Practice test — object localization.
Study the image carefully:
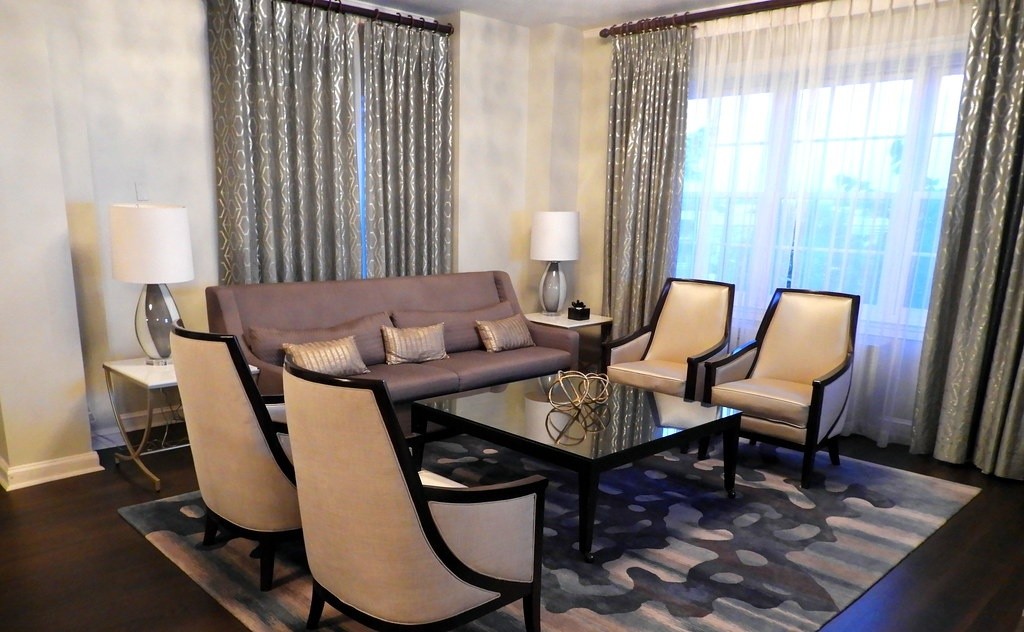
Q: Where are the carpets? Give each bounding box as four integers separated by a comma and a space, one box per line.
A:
115, 414, 984, 632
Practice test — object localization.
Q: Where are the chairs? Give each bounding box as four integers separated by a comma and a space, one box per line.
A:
282, 353, 549, 632
168, 322, 300, 594
698, 289, 861, 488
602, 277, 737, 455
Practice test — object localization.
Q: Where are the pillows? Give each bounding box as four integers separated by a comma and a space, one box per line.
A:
475, 312, 536, 354
377, 321, 451, 365
247, 310, 396, 372
278, 333, 372, 378
388, 300, 515, 351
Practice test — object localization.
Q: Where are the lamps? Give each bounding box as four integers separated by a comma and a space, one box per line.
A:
530, 210, 581, 317
110, 202, 196, 365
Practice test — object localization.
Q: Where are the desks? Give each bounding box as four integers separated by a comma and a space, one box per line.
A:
404, 368, 743, 556
524, 312, 614, 373
104, 357, 260, 491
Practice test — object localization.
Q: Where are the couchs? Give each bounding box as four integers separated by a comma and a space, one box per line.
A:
206, 269, 580, 445
